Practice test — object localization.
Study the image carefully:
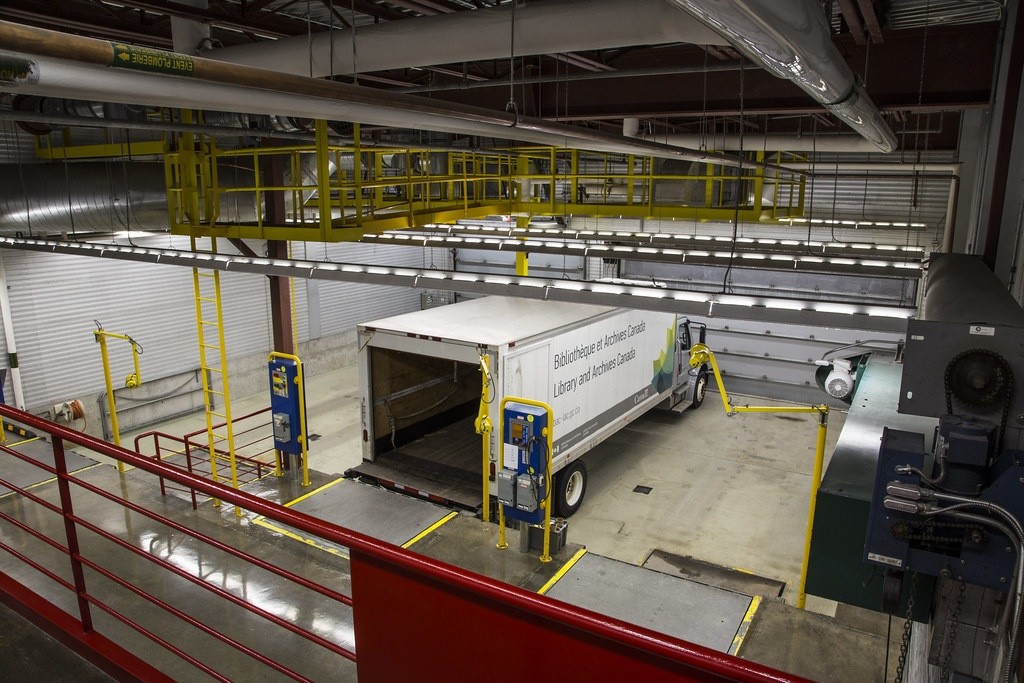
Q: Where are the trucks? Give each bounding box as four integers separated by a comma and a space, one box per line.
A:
344, 277, 709, 518
